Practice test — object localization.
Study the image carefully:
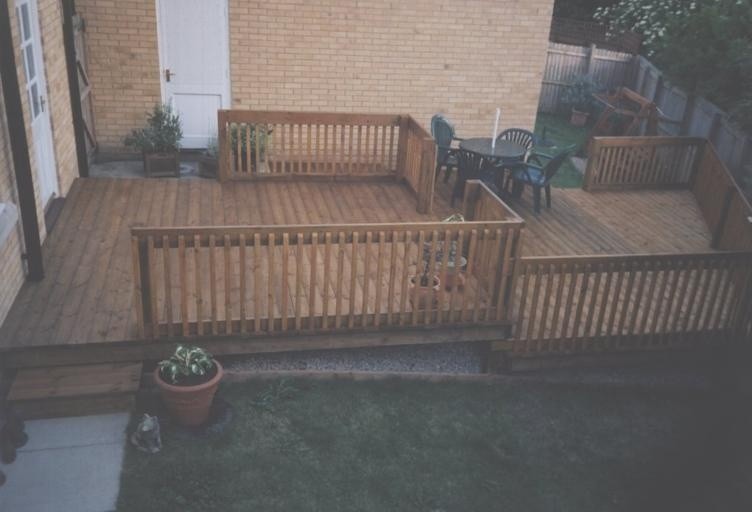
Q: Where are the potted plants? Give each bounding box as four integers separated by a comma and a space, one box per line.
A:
154, 346, 225, 429
122, 103, 274, 179
407, 241, 467, 309
559, 75, 605, 129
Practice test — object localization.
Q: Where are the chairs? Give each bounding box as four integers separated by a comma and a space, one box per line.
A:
430, 113, 577, 214
